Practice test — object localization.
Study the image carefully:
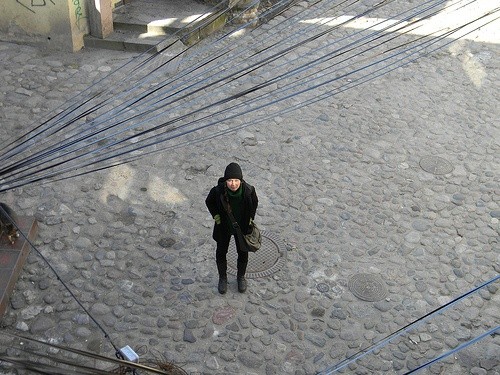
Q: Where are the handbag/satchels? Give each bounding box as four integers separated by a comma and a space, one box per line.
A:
243, 221, 262, 253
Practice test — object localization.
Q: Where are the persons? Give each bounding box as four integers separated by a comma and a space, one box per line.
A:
205, 162, 259, 295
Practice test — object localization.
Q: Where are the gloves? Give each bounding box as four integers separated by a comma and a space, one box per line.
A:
214, 215, 221, 225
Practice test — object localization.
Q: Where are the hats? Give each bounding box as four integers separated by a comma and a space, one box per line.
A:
224, 162, 243, 182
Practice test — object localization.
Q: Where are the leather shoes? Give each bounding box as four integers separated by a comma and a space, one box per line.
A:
237, 275, 248, 293
218, 274, 228, 295
